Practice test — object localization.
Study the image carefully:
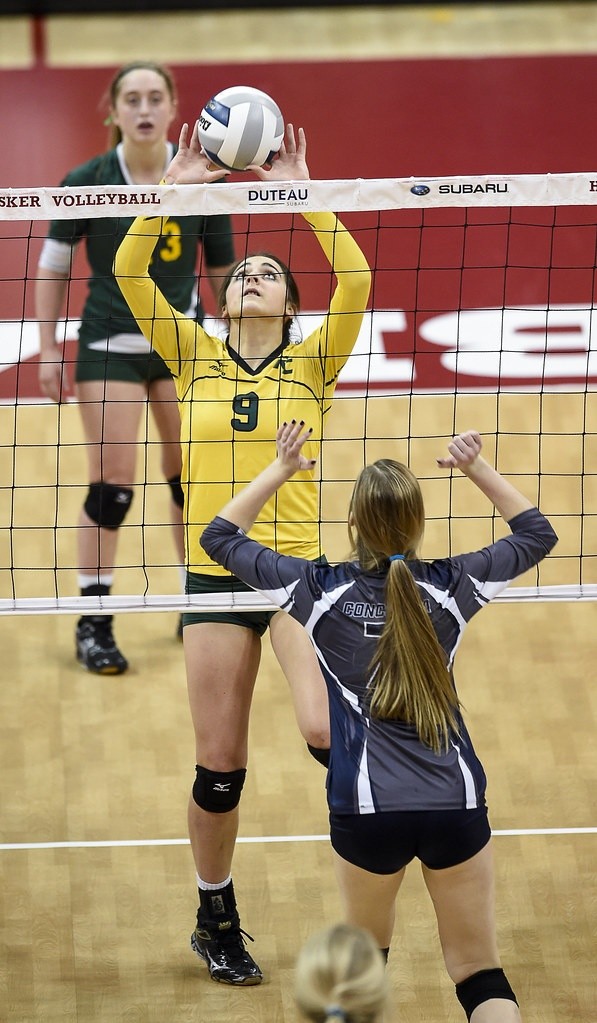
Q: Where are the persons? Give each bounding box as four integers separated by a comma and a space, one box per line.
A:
32, 58, 240, 678
200, 421, 560, 1023
288, 921, 391, 1023
111, 119, 372, 988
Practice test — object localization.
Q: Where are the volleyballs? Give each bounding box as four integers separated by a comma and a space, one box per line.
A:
196, 84, 287, 175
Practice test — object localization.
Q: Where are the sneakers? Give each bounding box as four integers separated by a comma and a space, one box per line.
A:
76, 618, 128, 675
190, 907, 263, 985
